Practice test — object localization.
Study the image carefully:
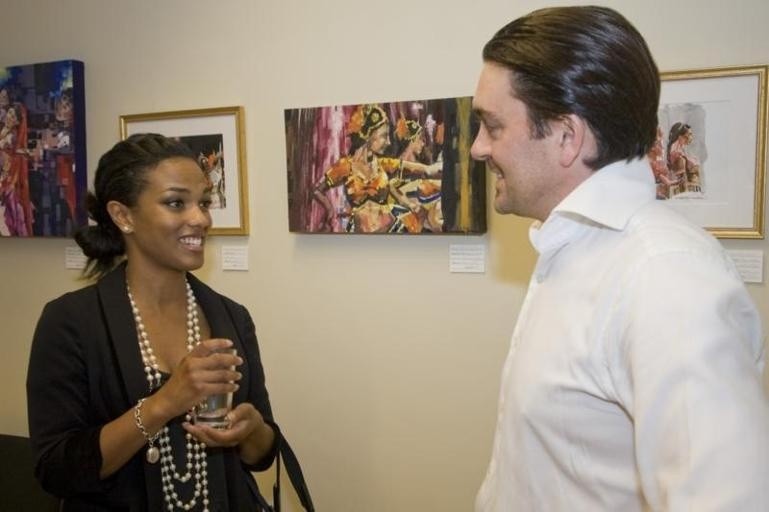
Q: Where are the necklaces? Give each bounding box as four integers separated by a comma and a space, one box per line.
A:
124, 275, 209, 511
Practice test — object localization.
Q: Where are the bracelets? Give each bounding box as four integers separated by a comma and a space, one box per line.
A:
135, 398, 165, 465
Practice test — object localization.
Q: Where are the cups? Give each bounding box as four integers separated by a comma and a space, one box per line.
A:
192, 348, 238, 431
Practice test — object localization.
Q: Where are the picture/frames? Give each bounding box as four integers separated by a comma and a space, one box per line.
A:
647, 65, 767, 237
119, 105, 249, 235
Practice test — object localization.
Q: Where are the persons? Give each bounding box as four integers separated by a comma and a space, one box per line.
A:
0, 82, 36, 236
312, 104, 444, 235
667, 122, 701, 195
26, 133, 281, 511
470, 6, 768, 512
387, 119, 443, 235
29, 87, 78, 237
